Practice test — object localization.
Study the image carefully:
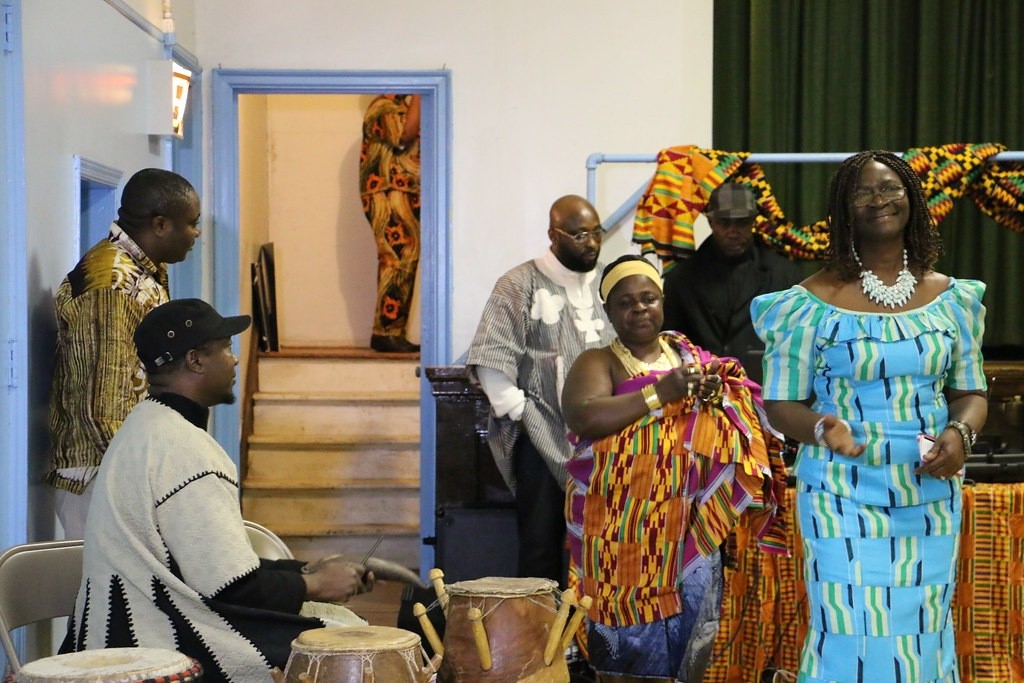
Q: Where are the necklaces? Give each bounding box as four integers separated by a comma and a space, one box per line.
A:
610, 336, 677, 377
851, 240, 918, 309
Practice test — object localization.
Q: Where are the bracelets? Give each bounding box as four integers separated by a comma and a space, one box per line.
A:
943, 420, 977, 460
641, 383, 662, 411
815, 417, 852, 447
713, 386, 724, 405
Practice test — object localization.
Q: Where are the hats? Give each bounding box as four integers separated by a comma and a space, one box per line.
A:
705, 184, 760, 220
136, 298, 251, 371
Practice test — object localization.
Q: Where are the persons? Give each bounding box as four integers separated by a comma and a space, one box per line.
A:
58, 298, 375, 682
663, 183, 785, 367
358, 94, 420, 353
466, 195, 619, 593
562, 255, 791, 683
749, 149, 988, 681
41, 167, 202, 541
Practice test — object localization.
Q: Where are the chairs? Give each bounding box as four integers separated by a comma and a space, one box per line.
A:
0, 540, 83, 669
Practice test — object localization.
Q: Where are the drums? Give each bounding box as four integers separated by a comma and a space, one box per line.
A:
443, 574, 572, 683
280, 623, 427, 683
6, 647, 206, 683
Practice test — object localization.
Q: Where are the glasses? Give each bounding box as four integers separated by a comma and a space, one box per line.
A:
552, 227, 608, 244
850, 187, 909, 207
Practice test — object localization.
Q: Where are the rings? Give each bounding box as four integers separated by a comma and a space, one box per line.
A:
687, 390, 691, 397
686, 363, 695, 374
702, 396, 710, 402
716, 375, 722, 384
688, 382, 693, 389
709, 390, 715, 397
